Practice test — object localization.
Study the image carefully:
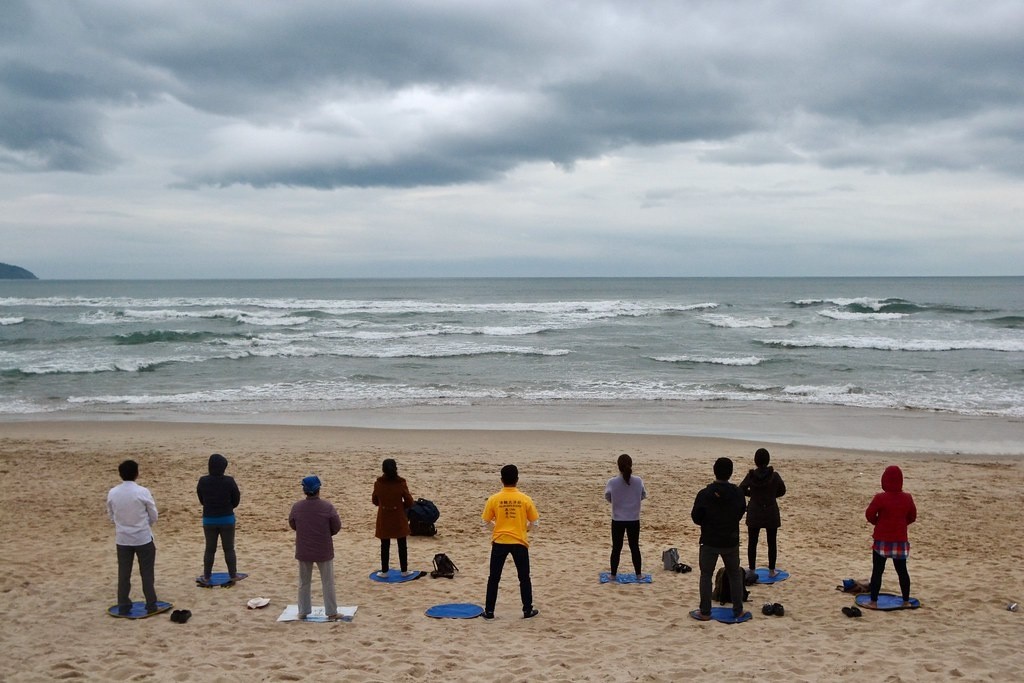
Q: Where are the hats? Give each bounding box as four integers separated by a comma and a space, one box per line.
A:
247, 597, 270, 608
303, 476, 321, 493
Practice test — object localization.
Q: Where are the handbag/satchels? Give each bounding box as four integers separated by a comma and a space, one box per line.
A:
837, 579, 871, 594
662, 547, 679, 570
432, 553, 458, 572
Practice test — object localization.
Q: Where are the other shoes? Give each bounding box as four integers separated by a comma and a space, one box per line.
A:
676, 564, 684, 572
431, 571, 437, 578
178, 610, 191, 623
441, 572, 454, 579
842, 607, 854, 617
682, 565, 692, 573
773, 603, 784, 616
762, 604, 773, 615
171, 610, 180, 621
851, 607, 862, 617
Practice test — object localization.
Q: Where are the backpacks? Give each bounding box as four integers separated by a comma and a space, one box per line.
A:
712, 567, 748, 602
410, 498, 439, 535
748, 467, 774, 511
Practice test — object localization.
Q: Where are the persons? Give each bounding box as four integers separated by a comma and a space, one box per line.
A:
862, 466, 917, 609
371, 459, 414, 578
604, 454, 647, 581
196, 455, 241, 583
480, 465, 538, 619
739, 448, 786, 579
106, 460, 160, 610
690, 458, 749, 620
288, 476, 344, 620
